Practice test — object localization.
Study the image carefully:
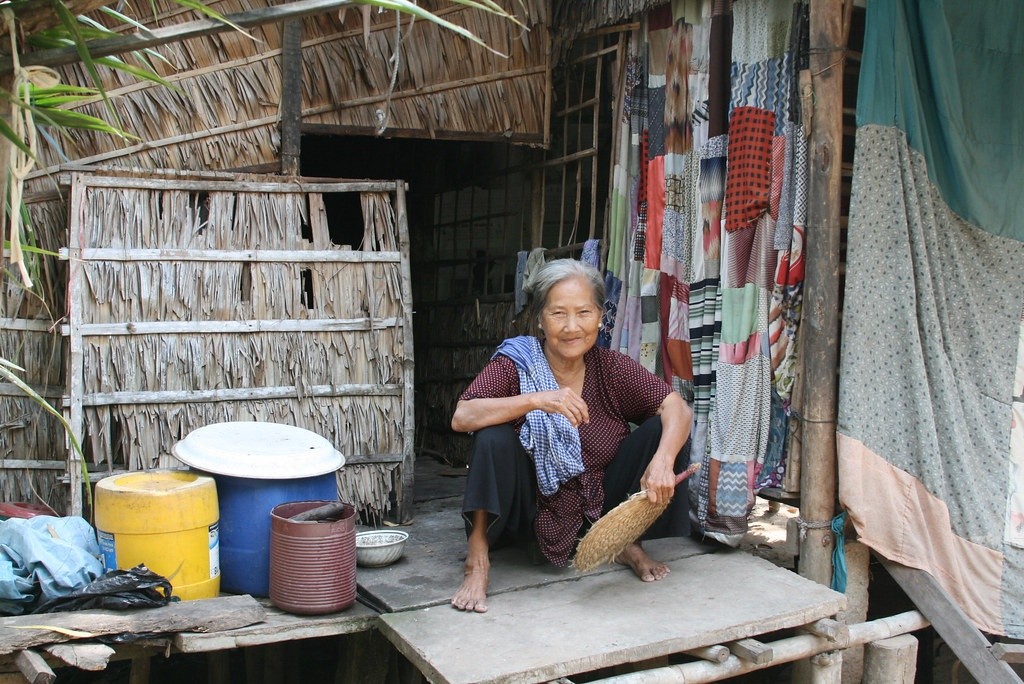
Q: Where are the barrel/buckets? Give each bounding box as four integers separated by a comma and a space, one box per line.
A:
93, 469, 221, 603
268, 499, 356, 614
189, 463, 339, 596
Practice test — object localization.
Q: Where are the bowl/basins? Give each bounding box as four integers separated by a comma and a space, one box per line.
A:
356, 530, 409, 567
172, 423, 346, 481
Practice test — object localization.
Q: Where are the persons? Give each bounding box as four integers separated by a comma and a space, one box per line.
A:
447, 257, 695, 615
